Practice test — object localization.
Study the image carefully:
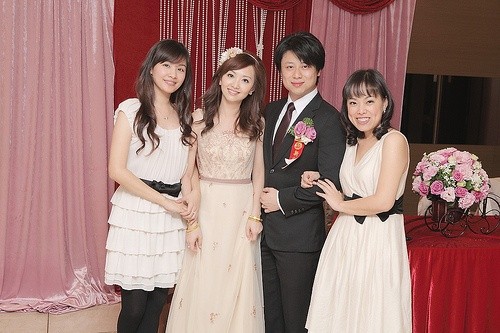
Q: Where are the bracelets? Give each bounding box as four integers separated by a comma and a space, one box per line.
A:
248, 216, 260, 222
186, 222, 199, 233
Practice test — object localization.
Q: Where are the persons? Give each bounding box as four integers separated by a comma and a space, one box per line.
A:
101, 39, 201, 333
301, 69, 413, 333
260, 32, 347, 333
164, 47, 265, 333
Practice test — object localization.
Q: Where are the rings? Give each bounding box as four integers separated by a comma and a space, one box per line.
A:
192, 211, 195, 213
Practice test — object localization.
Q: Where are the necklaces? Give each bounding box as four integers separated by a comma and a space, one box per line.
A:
155, 103, 173, 119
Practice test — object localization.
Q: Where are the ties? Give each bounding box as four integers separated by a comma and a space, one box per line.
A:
272, 102, 295, 163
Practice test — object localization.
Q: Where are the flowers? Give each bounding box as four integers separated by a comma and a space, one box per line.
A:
220, 47, 242, 66
412, 147, 490, 209
289, 118, 317, 145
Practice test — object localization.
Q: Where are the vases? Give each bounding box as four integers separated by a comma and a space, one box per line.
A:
424, 183, 500, 237
